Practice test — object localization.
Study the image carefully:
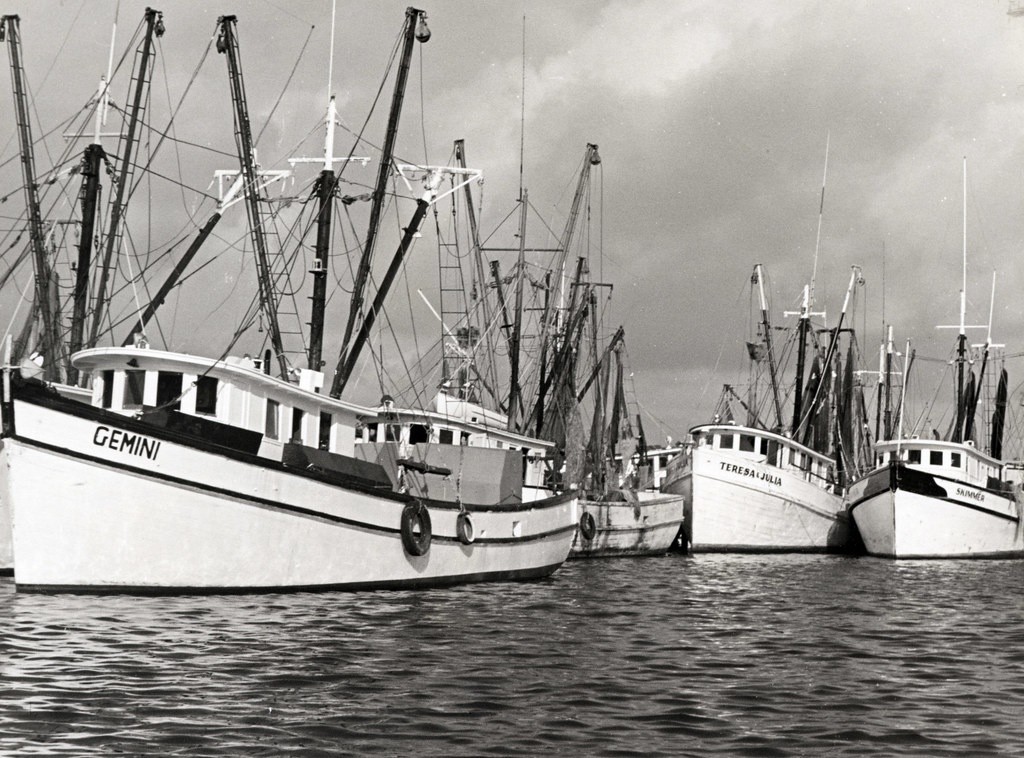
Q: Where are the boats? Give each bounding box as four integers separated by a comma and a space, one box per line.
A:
849, 440, 1024, 559
2, 347, 579, 595
566, 496, 685, 556
660, 424, 850, 551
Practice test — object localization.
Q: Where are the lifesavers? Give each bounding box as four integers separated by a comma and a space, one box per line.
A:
456, 512, 476, 545
579, 512, 596, 540
400, 500, 433, 555
